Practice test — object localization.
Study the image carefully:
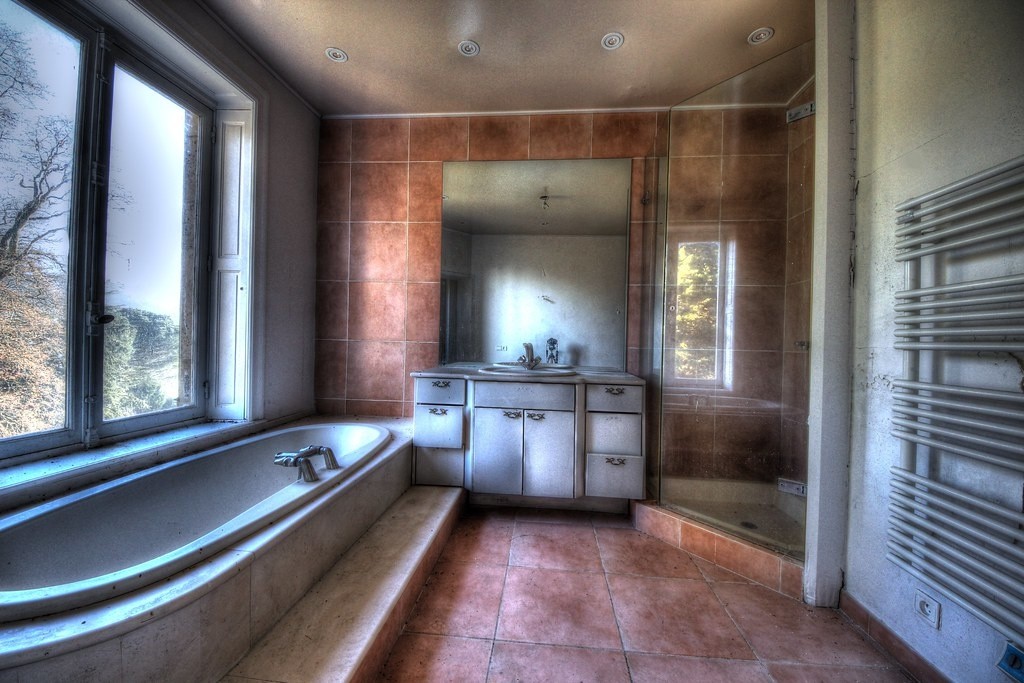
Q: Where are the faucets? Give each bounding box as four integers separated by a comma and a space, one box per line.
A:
272, 444, 341, 483
517, 342, 542, 370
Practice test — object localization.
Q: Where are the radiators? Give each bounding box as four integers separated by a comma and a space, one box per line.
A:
885, 157, 1024, 651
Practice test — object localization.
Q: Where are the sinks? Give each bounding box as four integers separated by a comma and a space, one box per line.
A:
478, 366, 577, 376
492, 361, 577, 368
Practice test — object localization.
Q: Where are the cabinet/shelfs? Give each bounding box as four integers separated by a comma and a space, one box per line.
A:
414, 378, 646, 500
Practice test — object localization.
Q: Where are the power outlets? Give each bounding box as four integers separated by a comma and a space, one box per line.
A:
913, 588, 940, 630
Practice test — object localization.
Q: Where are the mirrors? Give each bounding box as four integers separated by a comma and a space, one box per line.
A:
440, 158, 633, 372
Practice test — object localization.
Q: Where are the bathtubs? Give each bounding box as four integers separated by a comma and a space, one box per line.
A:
1, 419, 394, 619
